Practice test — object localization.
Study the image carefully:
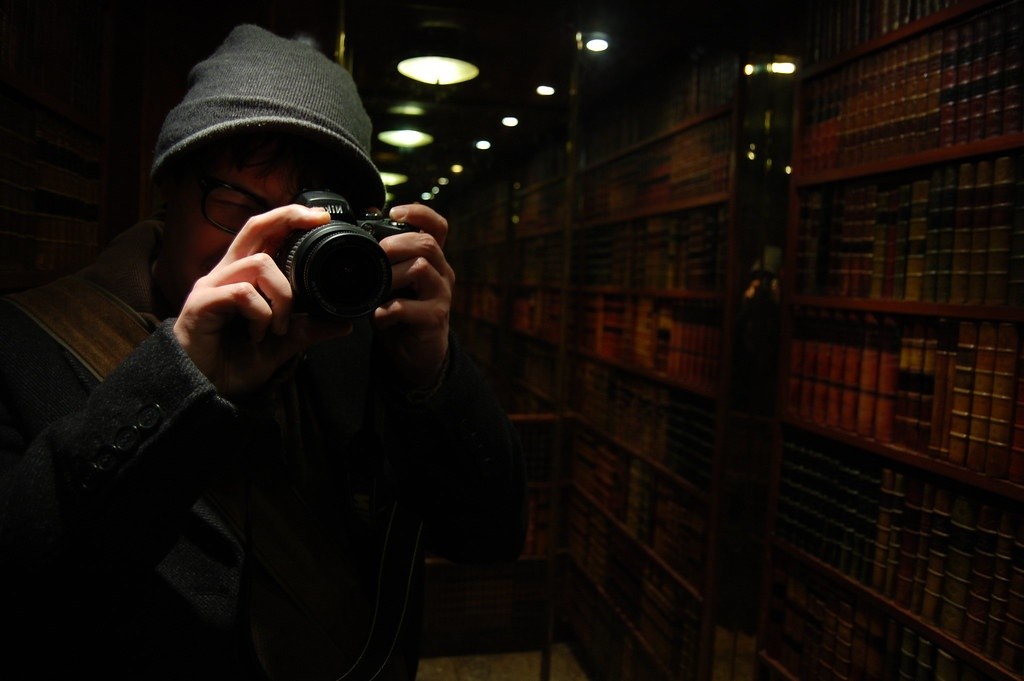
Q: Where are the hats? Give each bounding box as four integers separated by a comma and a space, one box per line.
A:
147, 24, 386, 215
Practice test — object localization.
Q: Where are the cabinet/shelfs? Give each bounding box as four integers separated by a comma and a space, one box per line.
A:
0, 0, 254, 305
420, 0, 1024, 681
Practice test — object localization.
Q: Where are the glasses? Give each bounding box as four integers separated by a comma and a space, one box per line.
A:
179, 180, 274, 238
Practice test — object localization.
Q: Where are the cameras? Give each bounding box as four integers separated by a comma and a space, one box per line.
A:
273, 187, 420, 325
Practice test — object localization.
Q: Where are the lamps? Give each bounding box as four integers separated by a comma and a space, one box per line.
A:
392, 13, 481, 88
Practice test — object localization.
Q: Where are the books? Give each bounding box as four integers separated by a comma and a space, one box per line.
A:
434, 0, 1024, 681
1, 0, 106, 293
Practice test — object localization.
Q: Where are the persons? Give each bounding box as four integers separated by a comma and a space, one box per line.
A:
0, 20, 529, 681
